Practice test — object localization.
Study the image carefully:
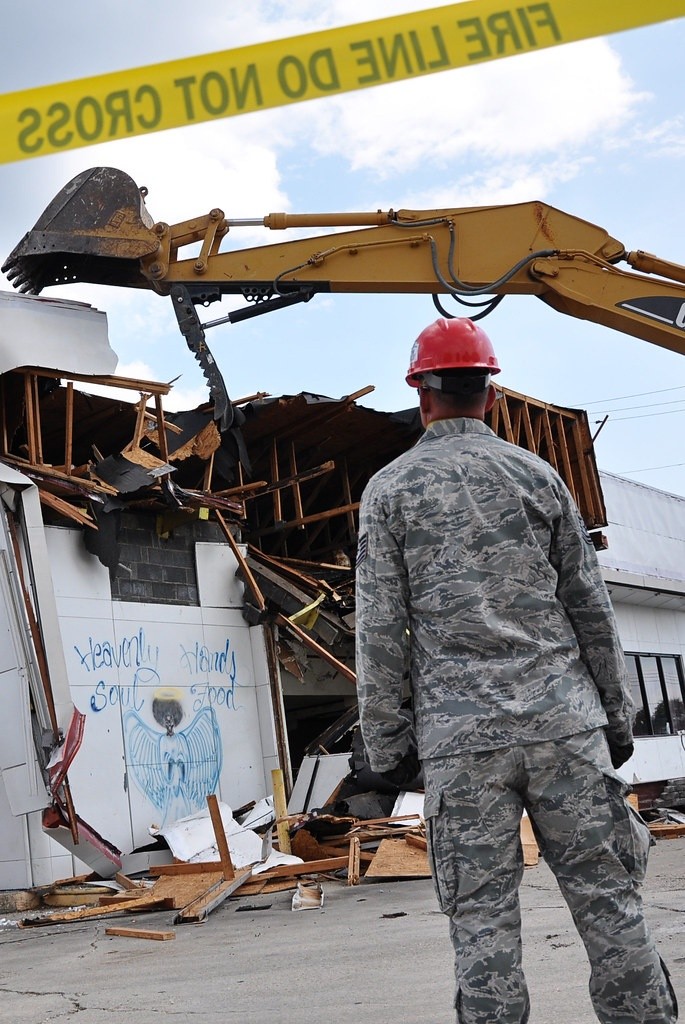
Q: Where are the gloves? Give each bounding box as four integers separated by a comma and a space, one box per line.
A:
378, 748, 421, 787
609, 740, 634, 768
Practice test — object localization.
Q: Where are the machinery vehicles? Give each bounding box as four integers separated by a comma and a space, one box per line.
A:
0, 164, 685, 438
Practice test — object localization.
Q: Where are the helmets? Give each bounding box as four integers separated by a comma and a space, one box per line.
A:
405, 317, 501, 387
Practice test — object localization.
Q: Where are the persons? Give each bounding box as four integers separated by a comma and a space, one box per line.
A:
355, 317, 679, 1024
